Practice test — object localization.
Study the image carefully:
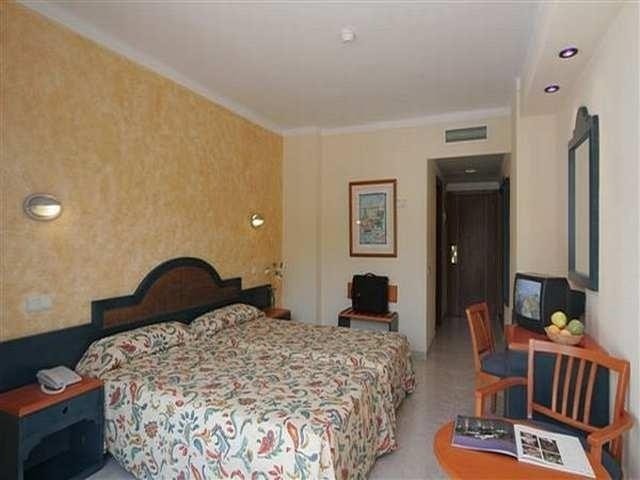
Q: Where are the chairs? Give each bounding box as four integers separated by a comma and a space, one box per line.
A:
474, 339, 634, 480
466, 303, 508, 417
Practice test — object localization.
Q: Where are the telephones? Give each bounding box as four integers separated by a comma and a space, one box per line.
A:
36, 365, 82, 394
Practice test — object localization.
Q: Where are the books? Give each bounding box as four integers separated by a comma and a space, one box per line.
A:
451, 413, 596, 479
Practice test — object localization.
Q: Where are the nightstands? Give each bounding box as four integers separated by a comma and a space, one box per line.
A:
0, 376, 106, 480
266, 308, 291, 321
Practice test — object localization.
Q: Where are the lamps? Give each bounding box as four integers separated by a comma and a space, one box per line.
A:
250, 212, 265, 229
23, 192, 63, 221
264, 255, 288, 309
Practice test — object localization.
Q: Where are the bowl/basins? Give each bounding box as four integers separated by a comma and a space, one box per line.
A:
544, 327, 584, 346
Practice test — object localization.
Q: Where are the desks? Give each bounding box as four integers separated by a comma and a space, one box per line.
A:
434, 414, 615, 480
338, 308, 399, 334
505, 324, 610, 453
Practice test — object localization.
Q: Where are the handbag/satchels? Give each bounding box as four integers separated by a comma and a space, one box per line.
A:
351, 272, 389, 314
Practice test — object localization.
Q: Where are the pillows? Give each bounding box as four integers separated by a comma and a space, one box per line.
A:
189, 303, 265, 341
75, 320, 198, 378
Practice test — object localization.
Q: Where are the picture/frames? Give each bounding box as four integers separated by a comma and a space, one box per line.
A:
349, 179, 398, 257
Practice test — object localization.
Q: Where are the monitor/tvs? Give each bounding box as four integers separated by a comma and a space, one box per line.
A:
513, 272, 586, 336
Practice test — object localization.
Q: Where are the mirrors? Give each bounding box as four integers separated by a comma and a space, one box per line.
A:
563, 106, 599, 293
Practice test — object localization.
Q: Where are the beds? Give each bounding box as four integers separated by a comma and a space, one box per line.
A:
211, 316, 416, 429
100, 338, 398, 480
